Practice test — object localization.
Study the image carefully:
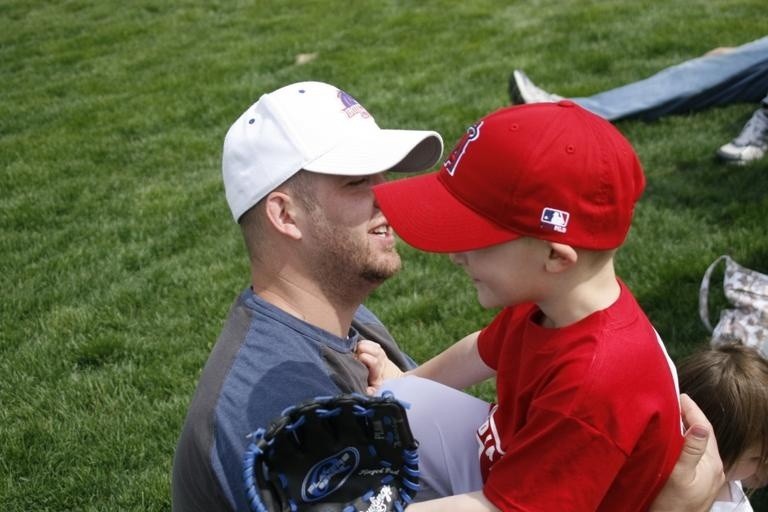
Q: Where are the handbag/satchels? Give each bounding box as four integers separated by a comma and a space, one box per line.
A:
711, 262, 768, 361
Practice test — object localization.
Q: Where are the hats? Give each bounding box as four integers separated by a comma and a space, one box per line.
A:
222, 81, 443, 224
372, 102, 646, 253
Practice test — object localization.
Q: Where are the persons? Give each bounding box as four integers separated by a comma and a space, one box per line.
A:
175, 79, 726, 511
675, 337, 768, 512
353, 99, 687, 512
509, 34, 768, 168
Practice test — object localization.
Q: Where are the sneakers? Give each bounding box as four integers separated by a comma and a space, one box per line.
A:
510, 70, 552, 103
716, 111, 768, 167
